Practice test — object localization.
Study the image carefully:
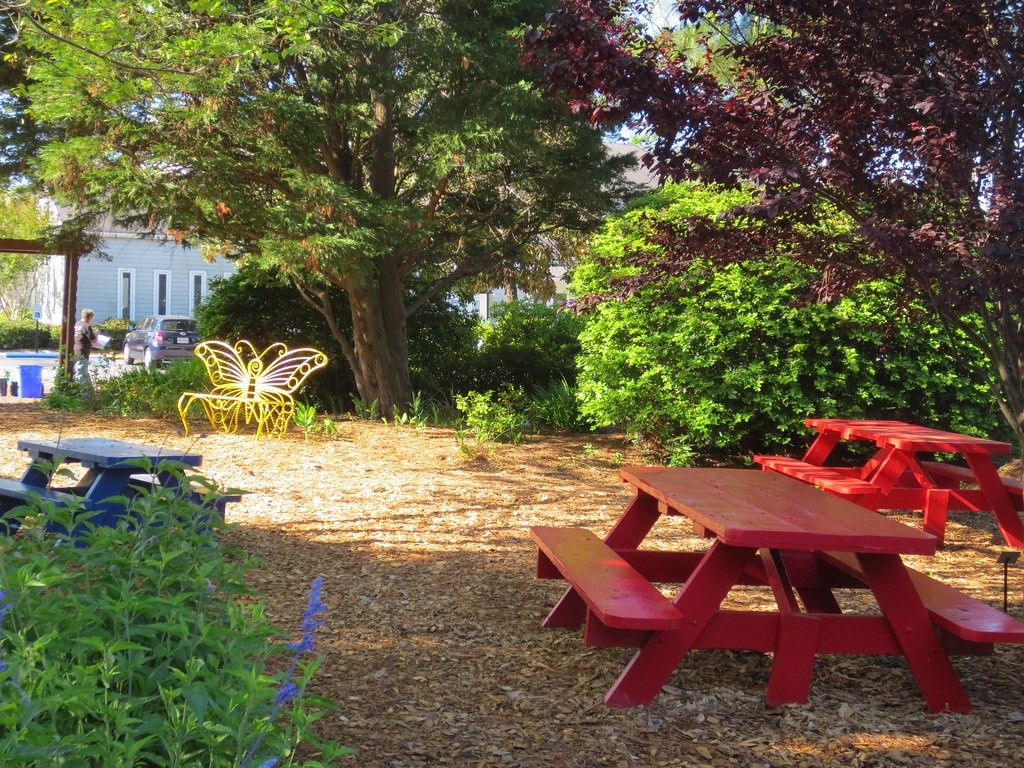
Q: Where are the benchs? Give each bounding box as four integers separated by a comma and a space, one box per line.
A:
920, 461, 1024, 497
129, 476, 242, 502
0, 479, 93, 512
814, 551, 1024, 643
529, 527, 684, 631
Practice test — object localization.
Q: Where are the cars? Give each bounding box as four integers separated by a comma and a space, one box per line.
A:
123, 315, 204, 371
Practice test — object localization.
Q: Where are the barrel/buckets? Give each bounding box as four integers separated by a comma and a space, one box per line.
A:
20, 365, 43, 398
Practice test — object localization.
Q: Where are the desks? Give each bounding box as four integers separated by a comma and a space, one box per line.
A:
754, 455, 882, 495
541, 467, 977, 717
802, 417, 1024, 550
0, 437, 225, 556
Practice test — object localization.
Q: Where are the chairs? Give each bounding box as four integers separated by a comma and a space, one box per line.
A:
178, 340, 329, 442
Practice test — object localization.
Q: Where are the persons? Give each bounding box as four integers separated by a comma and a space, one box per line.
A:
73, 308, 99, 367
